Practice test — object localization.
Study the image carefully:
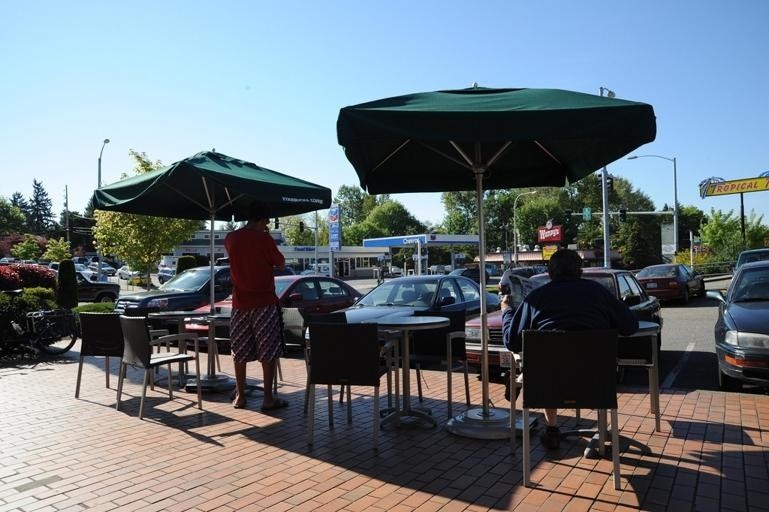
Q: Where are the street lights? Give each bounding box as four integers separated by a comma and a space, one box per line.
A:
98, 138, 110, 190
511, 191, 537, 263
628, 155, 679, 258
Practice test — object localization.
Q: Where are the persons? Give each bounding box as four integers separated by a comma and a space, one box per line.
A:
224, 200, 290, 410
501, 249, 639, 448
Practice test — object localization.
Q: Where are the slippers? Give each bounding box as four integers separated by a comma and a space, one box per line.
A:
261, 398, 289, 410
232, 396, 247, 408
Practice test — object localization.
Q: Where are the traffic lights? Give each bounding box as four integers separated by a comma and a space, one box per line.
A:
565, 210, 570, 222
300, 222, 304, 232
619, 209, 626, 222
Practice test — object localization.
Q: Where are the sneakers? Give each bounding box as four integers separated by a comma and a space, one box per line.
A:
540, 426, 559, 448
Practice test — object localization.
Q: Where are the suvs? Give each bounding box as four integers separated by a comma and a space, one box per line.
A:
157, 255, 210, 284
114, 264, 298, 347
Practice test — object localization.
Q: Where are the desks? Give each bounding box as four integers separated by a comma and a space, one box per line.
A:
359, 317, 451, 430
616, 320, 663, 435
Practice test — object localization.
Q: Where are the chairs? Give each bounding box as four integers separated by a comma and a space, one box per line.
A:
386, 309, 471, 418
298, 307, 400, 452
296, 309, 430, 429
104, 309, 287, 392
116, 312, 202, 420
75, 310, 154, 402
520, 327, 621, 491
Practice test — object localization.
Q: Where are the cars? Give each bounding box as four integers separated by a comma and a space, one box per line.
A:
183, 276, 362, 355
1, 256, 121, 305
633, 263, 706, 301
704, 260, 769, 395
464, 268, 665, 383
215, 257, 231, 265
391, 264, 490, 285
305, 275, 499, 370
732, 248, 769, 289
116, 265, 138, 280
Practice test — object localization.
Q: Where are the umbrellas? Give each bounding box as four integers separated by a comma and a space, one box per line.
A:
336, 82, 656, 413
93, 149, 331, 377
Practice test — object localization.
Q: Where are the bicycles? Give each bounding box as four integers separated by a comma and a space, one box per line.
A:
1, 289, 78, 357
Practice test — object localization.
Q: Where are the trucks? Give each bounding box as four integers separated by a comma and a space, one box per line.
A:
300, 263, 338, 278
